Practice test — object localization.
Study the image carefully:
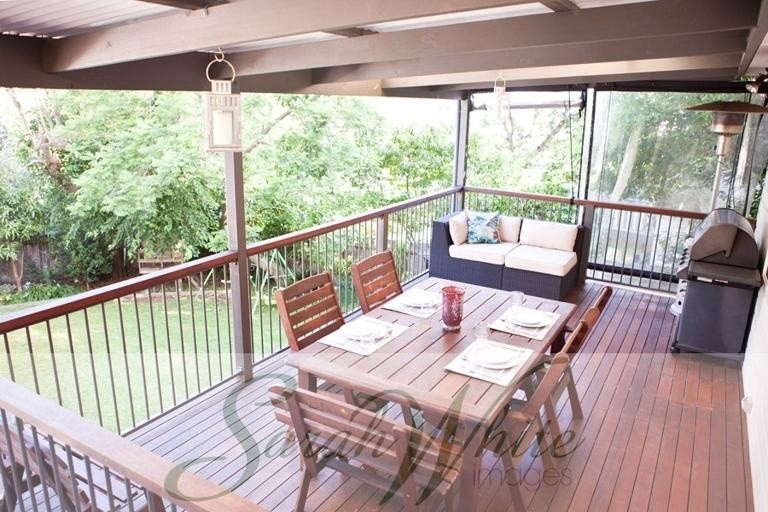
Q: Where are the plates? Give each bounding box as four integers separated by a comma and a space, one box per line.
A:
340, 321, 392, 342
502, 307, 552, 328
465, 346, 521, 370
399, 292, 442, 308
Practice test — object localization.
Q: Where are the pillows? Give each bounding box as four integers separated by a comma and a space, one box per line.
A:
466, 210, 501, 244
499, 215, 521, 243
449, 211, 468, 246
519, 218, 577, 250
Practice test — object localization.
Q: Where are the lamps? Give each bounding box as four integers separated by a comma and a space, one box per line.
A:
486, 74, 510, 130
202, 50, 243, 153
683, 100, 767, 210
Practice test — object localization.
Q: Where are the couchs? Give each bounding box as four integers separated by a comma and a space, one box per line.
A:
429, 210, 585, 301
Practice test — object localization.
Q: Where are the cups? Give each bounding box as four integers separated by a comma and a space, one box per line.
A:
440, 286, 466, 331
476, 321, 490, 340
510, 290, 524, 304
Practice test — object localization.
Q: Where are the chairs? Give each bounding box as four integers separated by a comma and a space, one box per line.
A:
276, 273, 415, 431
269, 385, 464, 512
1, 414, 164, 510
419, 319, 590, 512
516, 286, 613, 455
350, 249, 403, 313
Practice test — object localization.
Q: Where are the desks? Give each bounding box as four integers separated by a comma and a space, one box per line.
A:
286, 277, 577, 512
